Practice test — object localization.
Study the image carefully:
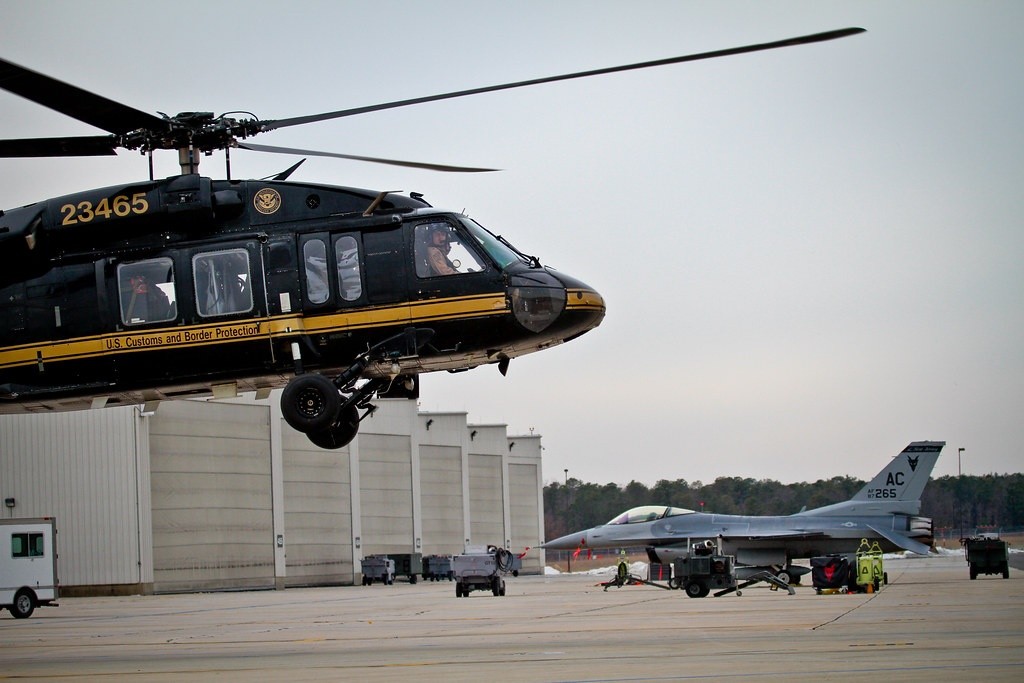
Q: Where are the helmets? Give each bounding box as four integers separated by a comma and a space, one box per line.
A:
130, 276, 150, 293
427, 222, 449, 247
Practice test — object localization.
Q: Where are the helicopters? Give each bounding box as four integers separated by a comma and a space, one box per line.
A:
0, 27, 864, 450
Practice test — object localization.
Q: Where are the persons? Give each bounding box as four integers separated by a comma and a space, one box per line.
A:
424, 225, 461, 275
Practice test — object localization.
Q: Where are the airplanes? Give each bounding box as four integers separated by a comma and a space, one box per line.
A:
533, 440, 947, 586
